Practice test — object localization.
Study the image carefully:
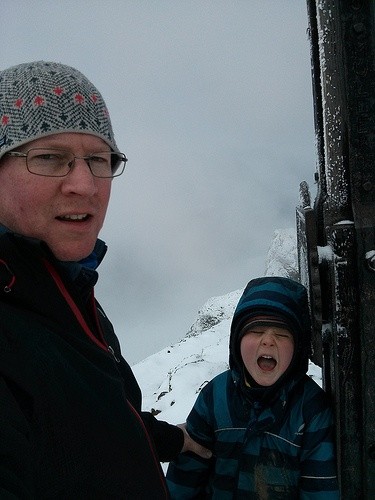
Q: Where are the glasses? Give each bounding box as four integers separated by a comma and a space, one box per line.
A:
5, 148, 128, 178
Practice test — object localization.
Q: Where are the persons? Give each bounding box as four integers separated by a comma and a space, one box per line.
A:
165, 276, 343, 500
1, 61, 211, 499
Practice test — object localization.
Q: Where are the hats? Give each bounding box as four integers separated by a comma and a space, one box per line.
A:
0, 60, 119, 160
240, 316, 291, 337
